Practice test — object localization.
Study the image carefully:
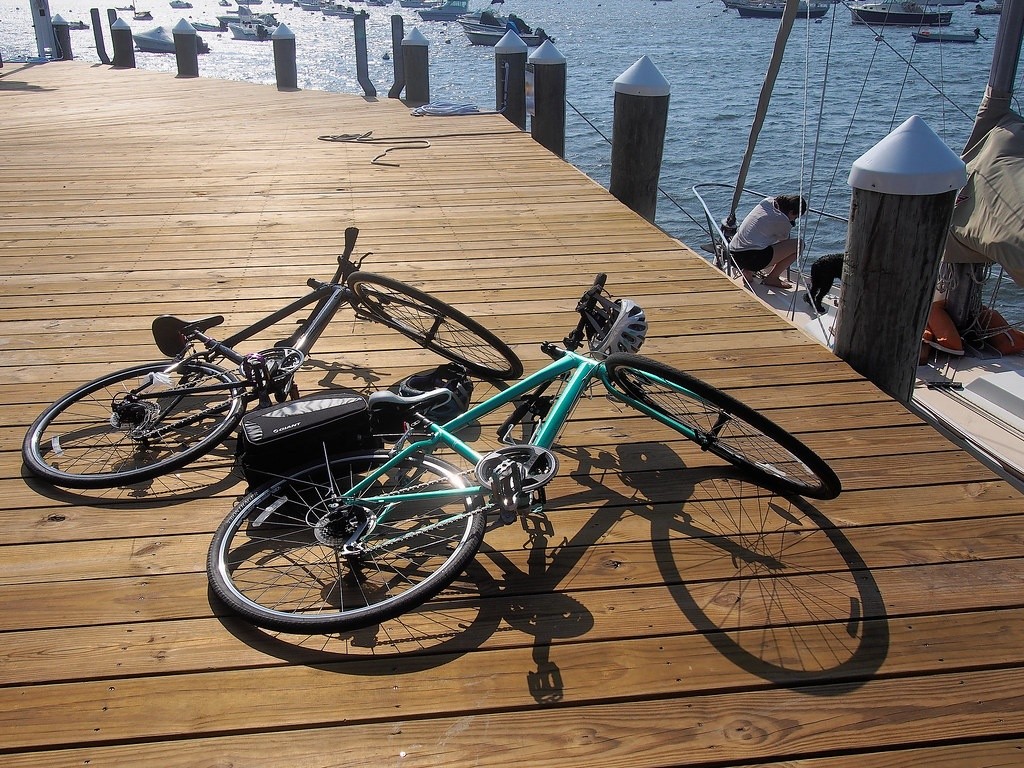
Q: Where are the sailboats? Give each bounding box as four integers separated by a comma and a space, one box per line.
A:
132, 0, 153, 20
690, 0, 1024, 499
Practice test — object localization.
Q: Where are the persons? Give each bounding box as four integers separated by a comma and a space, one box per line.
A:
728, 194, 806, 288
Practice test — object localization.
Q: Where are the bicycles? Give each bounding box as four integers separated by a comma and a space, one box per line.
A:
22, 226, 526, 491
208, 272, 842, 634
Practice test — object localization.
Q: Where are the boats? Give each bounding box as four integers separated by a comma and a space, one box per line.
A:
970, 0, 1004, 15
851, 0, 953, 26
190, 21, 229, 32
32, 19, 90, 30
911, 27, 980, 42
218, 0, 264, 6
273, 0, 355, 16
722, 0, 831, 18
339, 7, 370, 19
456, 0, 555, 46
215, 5, 281, 41
418, 0, 473, 21
115, 4, 135, 11
349, 0, 447, 8
131, 26, 211, 54
169, 0, 193, 9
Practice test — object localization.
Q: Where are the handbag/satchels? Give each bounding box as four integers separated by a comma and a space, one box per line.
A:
237, 394, 371, 507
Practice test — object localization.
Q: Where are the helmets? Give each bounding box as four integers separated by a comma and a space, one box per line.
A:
398, 363, 476, 427
585, 299, 648, 361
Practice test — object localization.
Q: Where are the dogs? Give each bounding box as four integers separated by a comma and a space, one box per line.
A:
802, 253, 844, 313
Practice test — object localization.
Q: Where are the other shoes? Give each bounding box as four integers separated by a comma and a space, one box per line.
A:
741, 274, 756, 282
766, 275, 792, 288
